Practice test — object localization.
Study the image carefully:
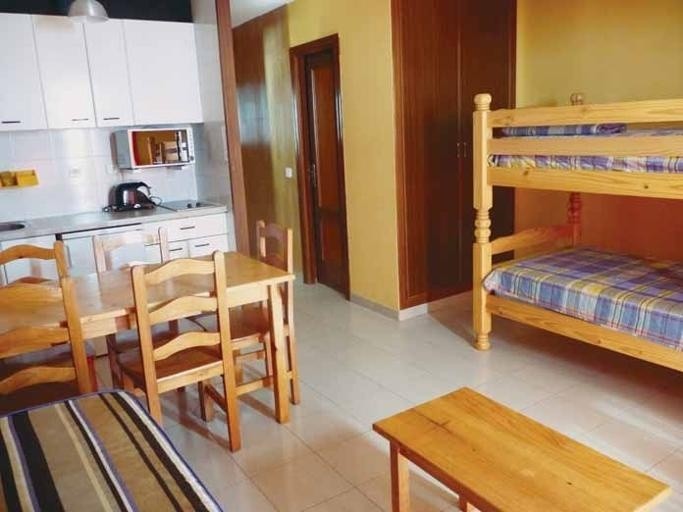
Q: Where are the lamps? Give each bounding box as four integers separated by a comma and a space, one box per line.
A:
68, 0, 108, 23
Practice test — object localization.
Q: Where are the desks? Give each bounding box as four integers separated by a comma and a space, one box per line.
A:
1, 389, 224, 512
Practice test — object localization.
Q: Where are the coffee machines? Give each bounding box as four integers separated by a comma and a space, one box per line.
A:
114, 181, 154, 211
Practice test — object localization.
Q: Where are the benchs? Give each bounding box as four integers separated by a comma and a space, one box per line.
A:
371, 386, 672, 511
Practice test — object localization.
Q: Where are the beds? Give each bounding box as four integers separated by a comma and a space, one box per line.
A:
469, 91, 682, 374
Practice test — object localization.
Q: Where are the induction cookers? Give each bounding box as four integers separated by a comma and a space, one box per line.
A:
160, 198, 214, 212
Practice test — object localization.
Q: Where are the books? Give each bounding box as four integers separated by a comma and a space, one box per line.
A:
131, 132, 189, 166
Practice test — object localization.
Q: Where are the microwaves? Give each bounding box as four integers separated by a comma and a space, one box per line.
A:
114, 126, 196, 171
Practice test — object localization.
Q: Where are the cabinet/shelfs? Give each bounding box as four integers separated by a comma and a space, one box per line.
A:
0, 212, 229, 285
389, 0, 518, 309
0, 12, 204, 131
